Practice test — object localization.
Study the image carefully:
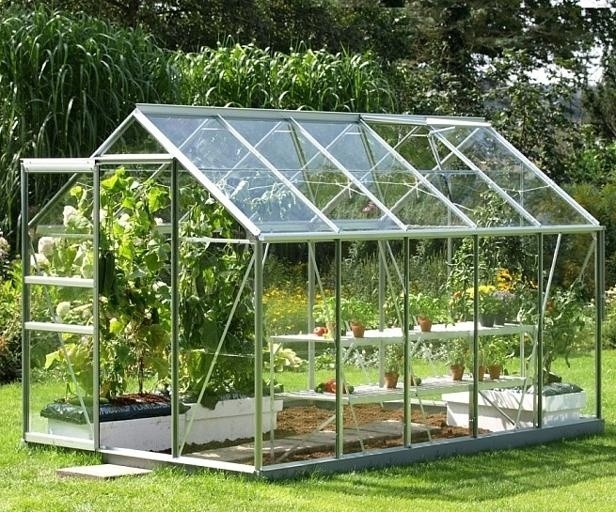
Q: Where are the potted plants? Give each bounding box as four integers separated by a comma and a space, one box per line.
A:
479, 344, 505, 381
450, 356, 466, 380
479, 290, 521, 328
384, 364, 400, 387
31, 165, 287, 452
342, 297, 376, 339
408, 293, 457, 332
